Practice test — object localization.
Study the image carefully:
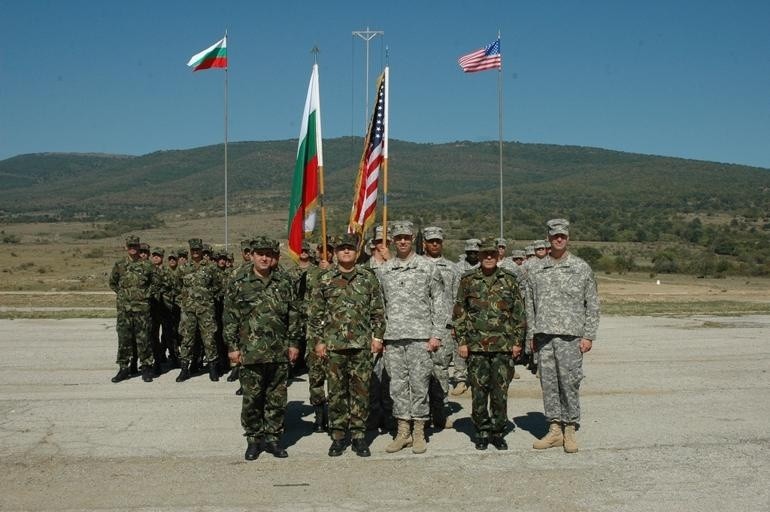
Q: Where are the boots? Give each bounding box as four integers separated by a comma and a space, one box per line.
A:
563, 425, 578, 453
433, 416, 454, 428
385, 419, 412, 453
451, 382, 468, 396
352, 439, 371, 457
533, 422, 564, 448
125, 235, 140, 244
413, 420, 427, 454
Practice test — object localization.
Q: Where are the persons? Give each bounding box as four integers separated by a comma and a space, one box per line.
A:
224, 232, 302, 460
176, 249, 189, 275
368, 238, 375, 257
512, 249, 523, 266
544, 241, 552, 254
287, 242, 314, 282
176, 238, 222, 382
310, 233, 386, 457
236, 236, 296, 396
211, 253, 223, 275
201, 244, 227, 291
494, 237, 526, 450
524, 246, 534, 260
526, 219, 600, 453
303, 241, 335, 430
362, 224, 395, 272
495, 238, 520, 379
227, 238, 252, 382
225, 252, 238, 269
109, 235, 161, 382
448, 239, 482, 396
424, 227, 461, 428
419, 238, 429, 258
216, 251, 226, 271
160, 252, 184, 362
314, 244, 321, 265
129, 243, 163, 375
375, 220, 449, 454
152, 247, 181, 372
525, 240, 546, 378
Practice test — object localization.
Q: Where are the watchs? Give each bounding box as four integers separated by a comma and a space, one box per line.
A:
373, 337, 384, 344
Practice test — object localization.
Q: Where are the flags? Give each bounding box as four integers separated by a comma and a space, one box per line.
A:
458, 40, 501, 73
348, 67, 388, 250
186, 35, 228, 72
289, 68, 324, 265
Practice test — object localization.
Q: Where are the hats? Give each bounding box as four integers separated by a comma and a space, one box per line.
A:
525, 246, 535, 256
249, 235, 276, 249
546, 219, 570, 237
424, 228, 444, 240
270, 239, 280, 255
318, 234, 335, 248
188, 238, 203, 251
152, 246, 165, 258
167, 251, 178, 259
480, 237, 496, 251
204, 242, 235, 267
494, 238, 507, 247
335, 233, 359, 248
393, 221, 414, 237
300, 241, 319, 260
534, 240, 545, 247
545, 239, 552, 248
177, 247, 188, 257
241, 238, 252, 253
512, 250, 525, 258
375, 226, 391, 240
138, 243, 150, 254
464, 239, 479, 252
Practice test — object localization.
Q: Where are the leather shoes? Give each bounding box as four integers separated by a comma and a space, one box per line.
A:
236, 388, 242, 395
311, 422, 323, 431
265, 442, 286, 457
476, 433, 488, 450
127, 367, 138, 376
210, 370, 219, 381
228, 371, 238, 381
329, 437, 350, 457
491, 436, 508, 450
143, 367, 153, 382
176, 369, 189, 382
111, 369, 128, 381
244, 442, 262, 459
